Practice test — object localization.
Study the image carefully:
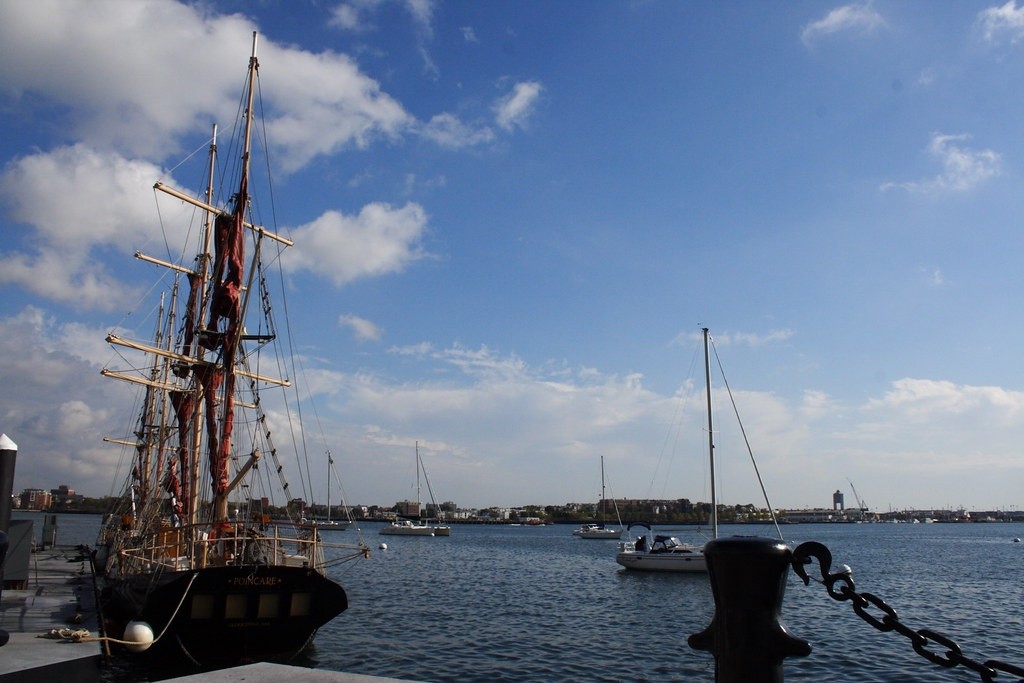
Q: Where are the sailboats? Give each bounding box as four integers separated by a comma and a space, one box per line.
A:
75, 29, 375, 683
614, 321, 784, 572
297, 449, 352, 530
571, 454, 624, 540
379, 440, 451, 537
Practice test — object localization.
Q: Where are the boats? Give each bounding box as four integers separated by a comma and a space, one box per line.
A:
776, 507, 862, 525
